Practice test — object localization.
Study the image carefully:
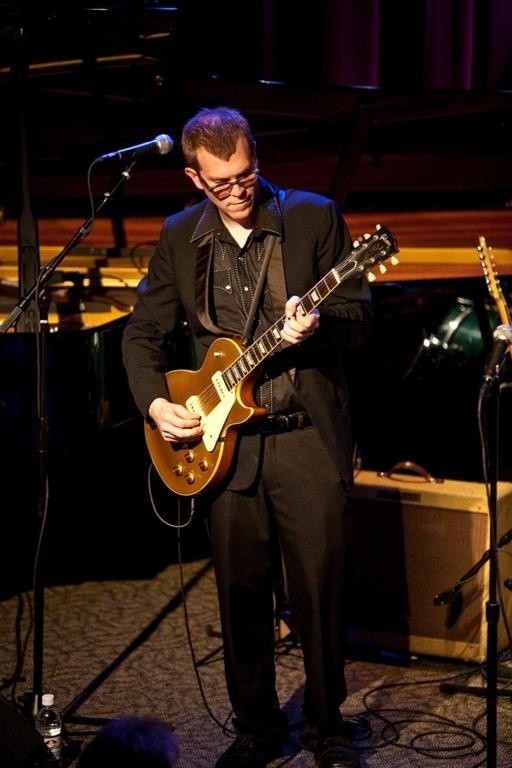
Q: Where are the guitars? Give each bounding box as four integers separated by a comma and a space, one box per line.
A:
141, 220, 396, 500
476, 236, 511, 329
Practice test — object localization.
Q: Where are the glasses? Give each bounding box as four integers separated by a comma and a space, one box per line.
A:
195, 168, 260, 197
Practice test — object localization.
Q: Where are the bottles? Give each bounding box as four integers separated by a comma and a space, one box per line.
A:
33, 692, 63, 766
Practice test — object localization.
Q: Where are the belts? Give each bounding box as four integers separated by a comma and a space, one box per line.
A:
261, 409, 313, 437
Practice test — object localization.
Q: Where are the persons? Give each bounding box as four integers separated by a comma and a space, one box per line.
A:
121, 106, 377, 768
77, 714, 180, 768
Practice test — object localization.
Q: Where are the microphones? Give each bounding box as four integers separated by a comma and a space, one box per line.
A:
480, 322, 512, 386
96, 133, 175, 163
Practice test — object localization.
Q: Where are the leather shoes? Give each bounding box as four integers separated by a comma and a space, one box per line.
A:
316, 735, 361, 767
214, 734, 285, 768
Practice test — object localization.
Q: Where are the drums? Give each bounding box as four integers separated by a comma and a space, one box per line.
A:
406, 298, 501, 482
